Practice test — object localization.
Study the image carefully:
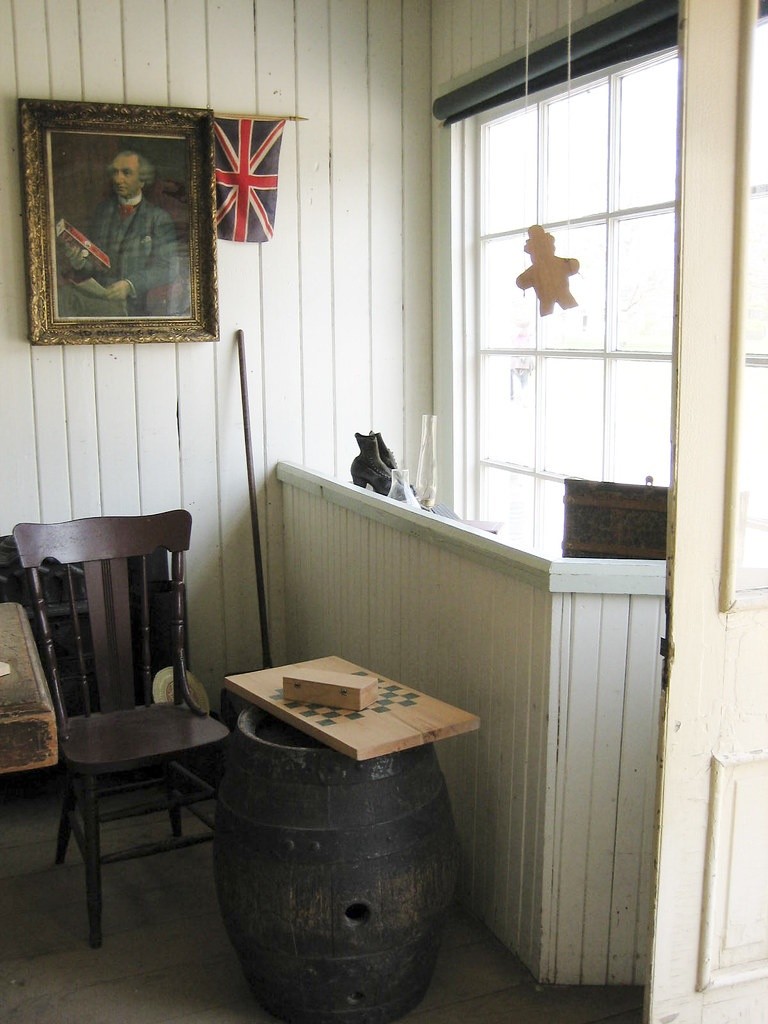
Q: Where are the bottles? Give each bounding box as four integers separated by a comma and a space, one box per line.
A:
414, 414, 439, 508
387, 468, 421, 510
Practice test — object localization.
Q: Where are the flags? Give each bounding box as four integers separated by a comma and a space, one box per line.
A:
214, 117, 284, 242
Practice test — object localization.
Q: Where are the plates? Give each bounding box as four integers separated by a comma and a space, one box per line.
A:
152, 666, 210, 717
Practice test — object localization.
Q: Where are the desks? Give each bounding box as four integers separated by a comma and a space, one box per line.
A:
11, 508, 230, 948
0, 602, 58, 775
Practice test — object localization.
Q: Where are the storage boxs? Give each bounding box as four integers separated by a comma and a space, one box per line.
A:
283, 668, 379, 711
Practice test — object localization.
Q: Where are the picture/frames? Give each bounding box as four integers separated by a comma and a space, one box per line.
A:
17, 97, 220, 345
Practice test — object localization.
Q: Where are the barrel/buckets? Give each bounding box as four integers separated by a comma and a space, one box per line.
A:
212, 705, 459, 1024
